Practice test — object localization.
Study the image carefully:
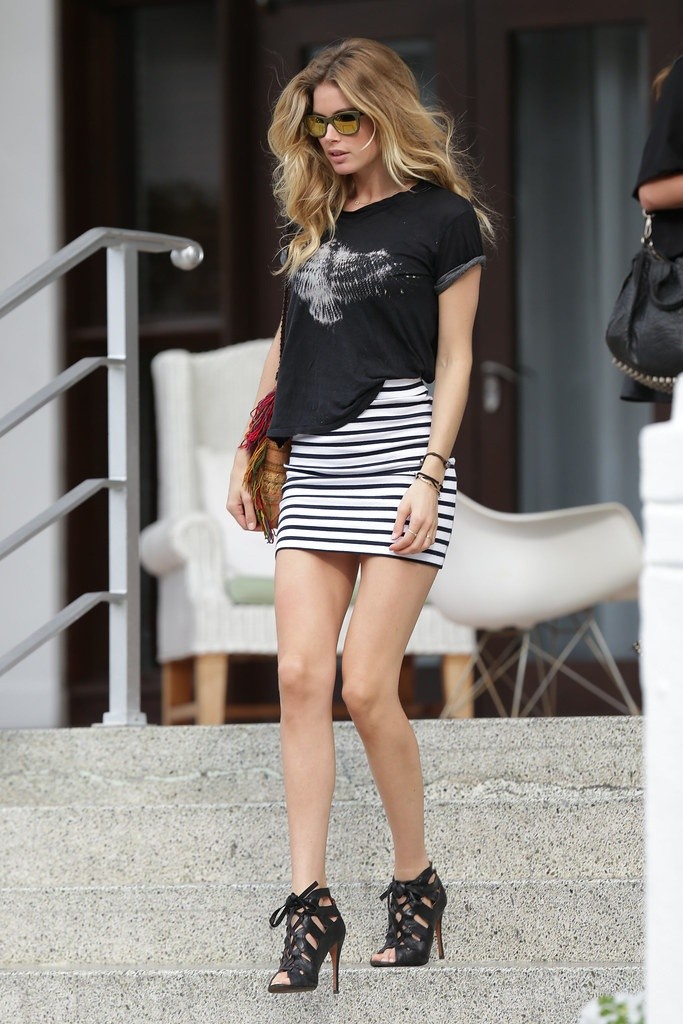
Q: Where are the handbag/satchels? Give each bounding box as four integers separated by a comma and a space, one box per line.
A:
239, 386, 292, 544
605, 208, 683, 395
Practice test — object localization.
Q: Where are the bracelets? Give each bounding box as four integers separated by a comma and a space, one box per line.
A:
421, 452, 451, 469
414, 471, 443, 496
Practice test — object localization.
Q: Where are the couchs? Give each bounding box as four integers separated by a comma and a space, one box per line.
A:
139, 335, 480, 728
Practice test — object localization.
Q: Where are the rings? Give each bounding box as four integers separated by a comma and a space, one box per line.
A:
407, 528, 417, 537
425, 534, 433, 540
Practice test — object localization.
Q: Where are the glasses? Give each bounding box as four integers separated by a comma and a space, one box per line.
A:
302, 109, 364, 138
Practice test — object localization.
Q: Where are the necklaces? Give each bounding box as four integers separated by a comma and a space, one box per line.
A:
348, 187, 404, 205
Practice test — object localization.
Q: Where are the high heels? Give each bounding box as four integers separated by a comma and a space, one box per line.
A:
267, 880, 346, 994
369, 861, 447, 967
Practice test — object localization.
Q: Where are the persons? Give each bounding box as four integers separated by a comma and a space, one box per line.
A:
622, 48, 682, 421
228, 36, 485, 995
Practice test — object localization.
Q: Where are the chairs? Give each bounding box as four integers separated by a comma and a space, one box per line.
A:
430, 489, 643, 716
